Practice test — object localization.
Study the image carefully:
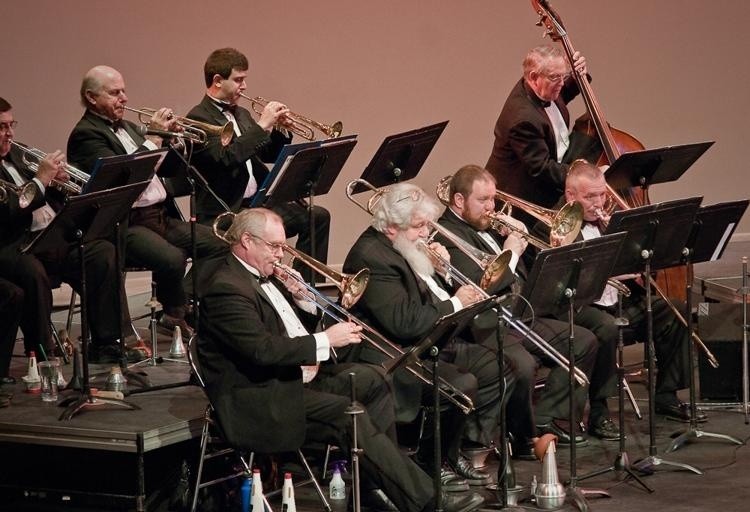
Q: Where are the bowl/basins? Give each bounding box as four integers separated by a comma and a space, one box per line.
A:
485, 480, 530, 503
22, 375, 41, 391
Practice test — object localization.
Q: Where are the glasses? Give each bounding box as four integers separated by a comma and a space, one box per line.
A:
248, 232, 288, 253
396, 188, 428, 206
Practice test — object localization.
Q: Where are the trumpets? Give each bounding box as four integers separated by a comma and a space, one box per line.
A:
119, 105, 234, 147
436, 174, 632, 298
8, 139, 91, 195
239, 91, 343, 141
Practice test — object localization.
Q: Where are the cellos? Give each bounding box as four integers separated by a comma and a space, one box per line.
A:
532, 0, 695, 310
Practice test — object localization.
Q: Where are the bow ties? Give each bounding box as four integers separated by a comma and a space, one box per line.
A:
208, 96, 238, 115
96, 116, 123, 133
580, 220, 600, 230
543, 100, 552, 110
258, 275, 272, 287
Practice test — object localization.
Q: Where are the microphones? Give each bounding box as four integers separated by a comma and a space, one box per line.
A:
135, 126, 188, 138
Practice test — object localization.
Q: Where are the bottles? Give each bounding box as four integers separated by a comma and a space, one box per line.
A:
329, 464, 345, 500
534, 441, 567, 509
242, 469, 265, 512
169, 326, 187, 358
27, 351, 39, 380
104, 367, 128, 393
282, 473, 297, 512
497, 438, 516, 488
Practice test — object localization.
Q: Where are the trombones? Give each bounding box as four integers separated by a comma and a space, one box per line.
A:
212, 211, 476, 414
568, 158, 720, 371
346, 178, 590, 388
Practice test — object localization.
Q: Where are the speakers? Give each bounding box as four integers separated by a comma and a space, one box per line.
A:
698, 303, 750, 399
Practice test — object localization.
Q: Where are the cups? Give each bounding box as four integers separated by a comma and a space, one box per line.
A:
38, 360, 60, 402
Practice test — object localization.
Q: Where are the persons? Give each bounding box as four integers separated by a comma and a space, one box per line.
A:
529, 162, 709, 442
180, 47, 330, 285
66, 65, 232, 341
0, 97, 144, 390
341, 182, 517, 494
197, 207, 485, 512
427, 164, 598, 460
480, 43, 611, 282
0, 251, 54, 391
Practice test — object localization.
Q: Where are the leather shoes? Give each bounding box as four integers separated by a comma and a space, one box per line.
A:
654, 398, 710, 422
435, 492, 486, 512
446, 450, 495, 485
148, 308, 198, 343
84, 339, 152, 369
536, 420, 589, 448
587, 418, 629, 441
413, 453, 469, 495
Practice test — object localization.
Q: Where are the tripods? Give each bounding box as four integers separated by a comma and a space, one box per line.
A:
564, 292, 654, 493
664, 259, 744, 453
125, 269, 194, 370
89, 222, 154, 389
125, 170, 209, 397
522, 297, 612, 512
58, 241, 143, 422
623, 261, 704, 481
